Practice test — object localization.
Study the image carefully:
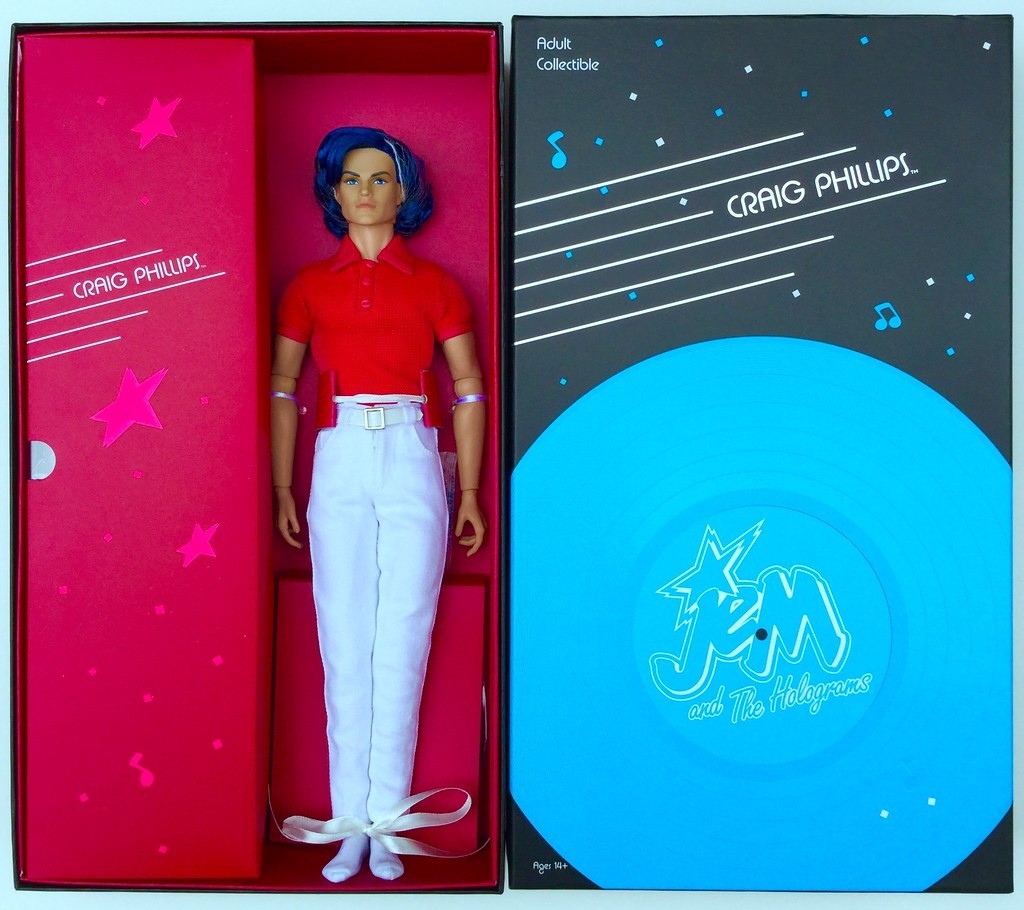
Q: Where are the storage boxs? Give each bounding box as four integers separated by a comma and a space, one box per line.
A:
9, 14, 1013, 897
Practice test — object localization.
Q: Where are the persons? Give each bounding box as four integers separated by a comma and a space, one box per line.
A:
271, 126, 484, 883
